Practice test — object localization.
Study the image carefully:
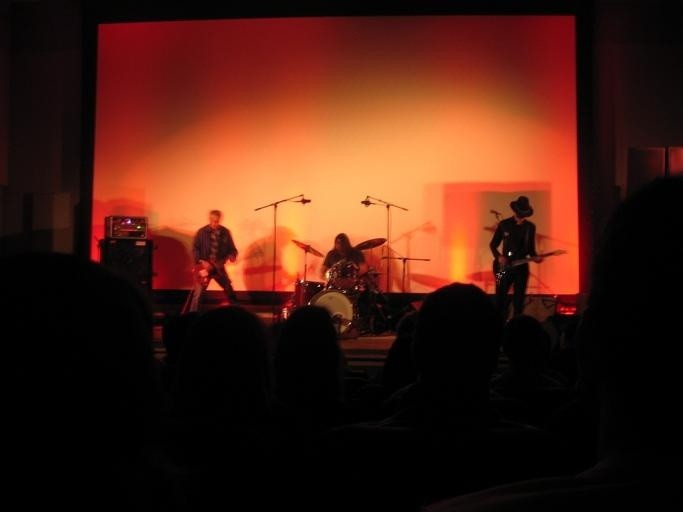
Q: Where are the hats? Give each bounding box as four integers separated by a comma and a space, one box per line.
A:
510, 196, 532, 217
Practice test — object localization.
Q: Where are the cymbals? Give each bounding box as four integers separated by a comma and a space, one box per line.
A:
292, 240, 326, 258
354, 239, 386, 250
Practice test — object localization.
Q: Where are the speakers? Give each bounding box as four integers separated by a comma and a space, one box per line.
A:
102, 239, 152, 295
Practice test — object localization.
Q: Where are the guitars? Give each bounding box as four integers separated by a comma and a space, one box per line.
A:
493, 250, 567, 281
193, 246, 263, 284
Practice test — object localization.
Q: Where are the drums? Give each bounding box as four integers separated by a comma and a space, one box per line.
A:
327, 260, 360, 290
294, 280, 325, 311
308, 287, 360, 338
358, 271, 381, 291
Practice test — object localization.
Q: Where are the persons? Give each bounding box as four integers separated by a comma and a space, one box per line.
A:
489, 197, 545, 320
2, 251, 606, 510
321, 235, 375, 334
190, 209, 237, 312
581, 175, 682, 509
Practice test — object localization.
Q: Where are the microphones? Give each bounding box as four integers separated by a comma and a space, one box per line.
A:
361, 200, 373, 207
300, 198, 311, 203
490, 208, 502, 216
